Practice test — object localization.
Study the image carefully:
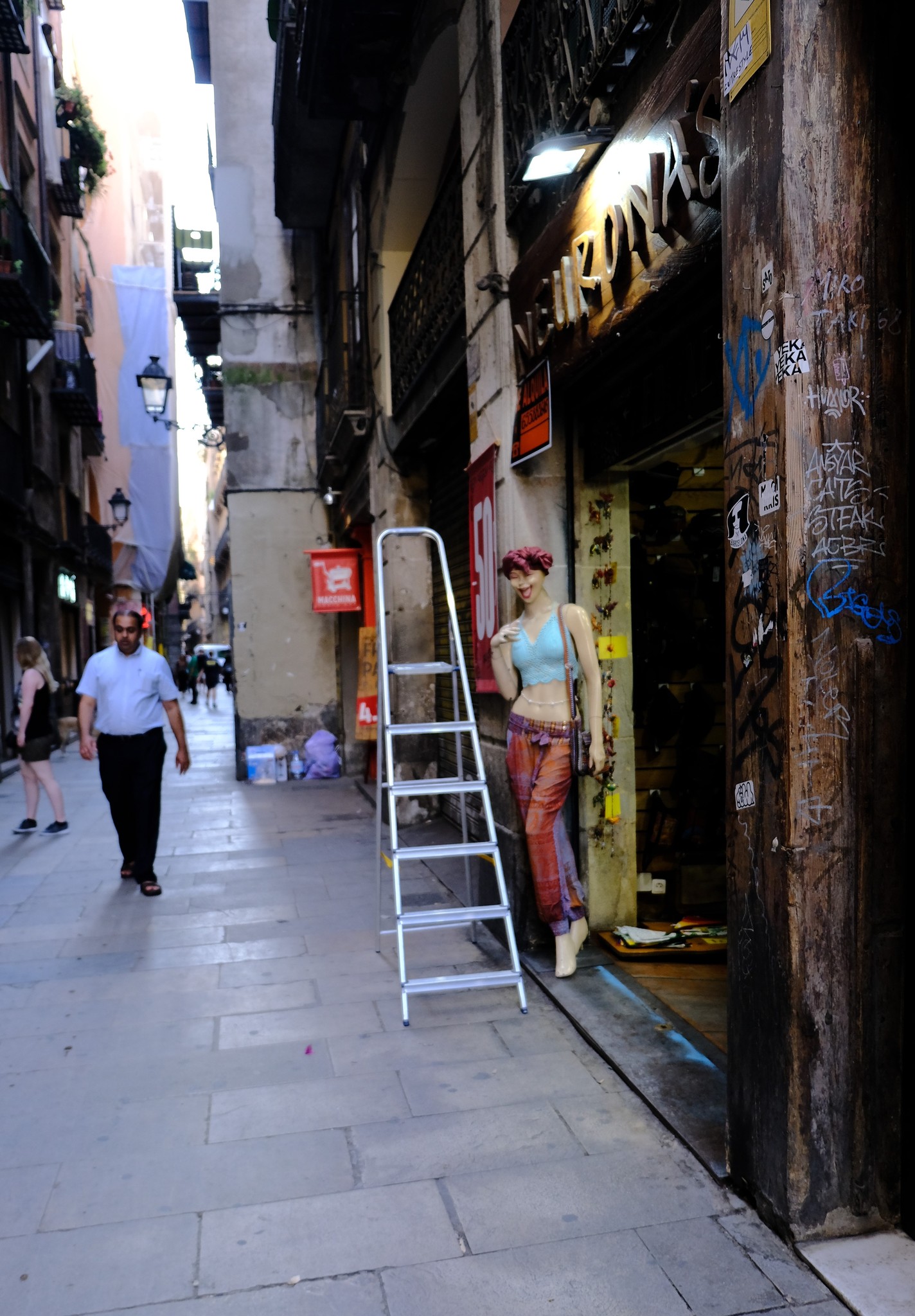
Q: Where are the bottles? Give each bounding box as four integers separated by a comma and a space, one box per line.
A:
291, 750, 302, 780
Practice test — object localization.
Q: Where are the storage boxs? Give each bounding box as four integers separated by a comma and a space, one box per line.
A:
245, 744, 289, 784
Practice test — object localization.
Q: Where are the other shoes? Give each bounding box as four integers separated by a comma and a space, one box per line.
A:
12, 818, 37, 832
40, 820, 70, 836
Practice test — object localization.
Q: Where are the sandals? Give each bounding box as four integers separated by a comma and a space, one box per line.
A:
120, 858, 136, 879
140, 880, 161, 896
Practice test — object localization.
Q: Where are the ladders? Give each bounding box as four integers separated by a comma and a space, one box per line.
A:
373, 523, 531, 1028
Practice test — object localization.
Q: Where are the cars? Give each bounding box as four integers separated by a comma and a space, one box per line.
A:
193, 644, 231, 661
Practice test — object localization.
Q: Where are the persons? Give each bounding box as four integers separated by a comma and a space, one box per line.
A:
491, 547, 607, 976
188, 649, 199, 705
75, 609, 190, 896
220, 650, 233, 695
196, 650, 221, 708
185, 650, 192, 663
12, 636, 69, 835
175, 656, 189, 700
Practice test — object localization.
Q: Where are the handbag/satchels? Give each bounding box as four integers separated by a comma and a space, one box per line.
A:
569, 726, 611, 777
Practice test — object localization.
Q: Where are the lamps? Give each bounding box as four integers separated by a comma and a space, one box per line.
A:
512, 128, 614, 187
135, 355, 225, 451
103, 487, 131, 530
